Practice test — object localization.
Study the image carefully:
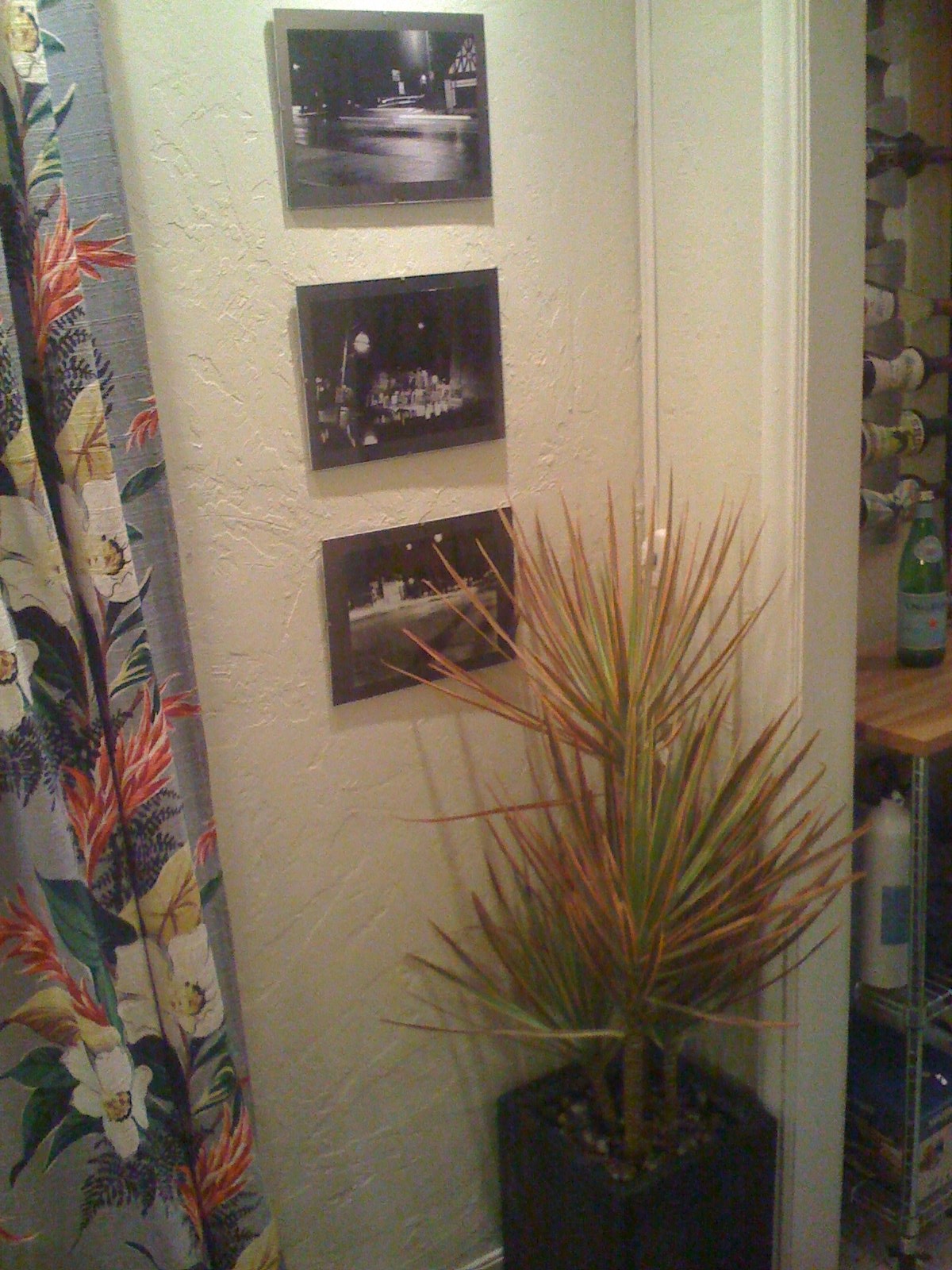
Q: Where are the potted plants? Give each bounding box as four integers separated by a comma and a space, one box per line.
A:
377, 462, 872, 1270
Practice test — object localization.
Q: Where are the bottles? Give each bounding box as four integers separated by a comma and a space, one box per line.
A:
860, 125, 951, 528
895, 488, 952, 668
859, 787, 914, 990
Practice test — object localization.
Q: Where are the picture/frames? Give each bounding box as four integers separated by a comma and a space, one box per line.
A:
272, 6, 492, 208
296, 266, 506, 469
320, 506, 516, 708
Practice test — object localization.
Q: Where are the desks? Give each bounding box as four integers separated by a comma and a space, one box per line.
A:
841, 618, 952, 1270
